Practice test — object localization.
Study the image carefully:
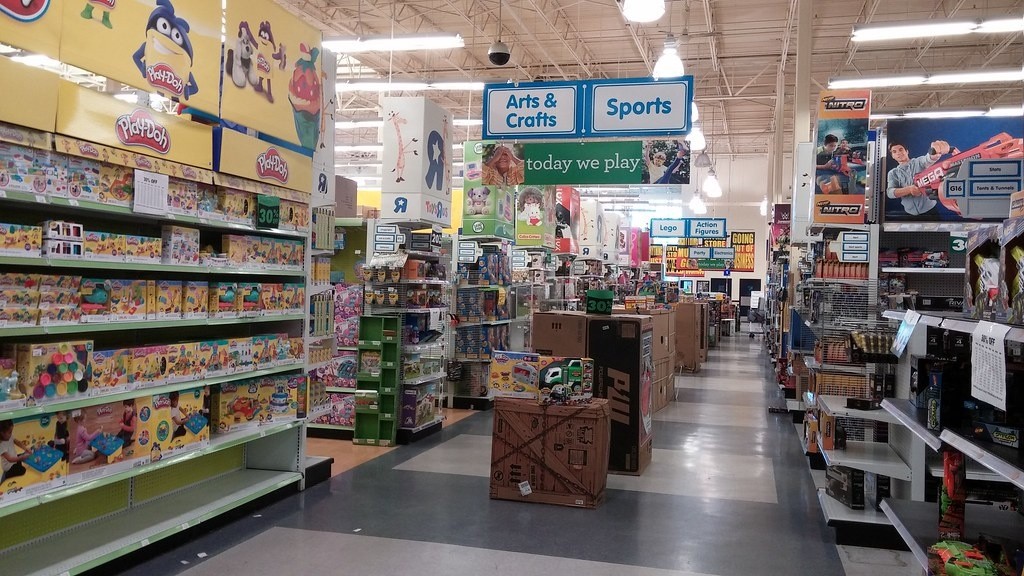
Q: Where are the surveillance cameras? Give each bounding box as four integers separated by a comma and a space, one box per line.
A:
487, 43, 510, 65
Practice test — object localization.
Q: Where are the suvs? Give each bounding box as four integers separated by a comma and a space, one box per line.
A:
542, 365, 583, 393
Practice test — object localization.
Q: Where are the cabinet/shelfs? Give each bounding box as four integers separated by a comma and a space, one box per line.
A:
0, 130, 310, 576
453, 238, 510, 400
354, 312, 401, 446
880, 223, 999, 314
372, 218, 453, 435
814, 305, 1008, 526
512, 250, 641, 352
881, 319, 1024, 576
764, 222, 876, 528
311, 219, 373, 430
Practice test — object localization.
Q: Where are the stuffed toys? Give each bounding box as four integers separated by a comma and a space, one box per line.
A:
227, 26, 259, 87
466, 186, 490, 215
519, 187, 545, 227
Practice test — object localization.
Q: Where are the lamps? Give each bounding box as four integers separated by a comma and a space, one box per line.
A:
321, 31, 512, 153
687, 42, 722, 213
654, 5, 686, 79
760, 154, 773, 216
828, 13, 1024, 120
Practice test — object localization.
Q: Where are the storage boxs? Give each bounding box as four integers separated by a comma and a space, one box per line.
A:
490, 399, 613, 509
908, 325, 1024, 450
455, 325, 510, 359
538, 356, 594, 406
0, 144, 310, 495
488, 350, 538, 400
868, 374, 883, 399
962, 192, 1024, 326
458, 253, 512, 399
820, 408, 835, 450
800, 411, 820, 453
625, 281, 737, 374
822, 465, 865, 510
866, 471, 893, 512
478, 254, 512, 286
901, 295, 965, 312
381, 96, 651, 270
808, 369, 866, 398
310, 282, 365, 426
532, 310, 654, 476
399, 261, 450, 429
883, 374, 897, 399
611, 306, 676, 414
332, 175, 357, 218
845, 398, 883, 411
0, 54, 314, 194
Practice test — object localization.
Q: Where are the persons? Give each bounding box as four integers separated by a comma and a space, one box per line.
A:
482, 146, 525, 186
0, 390, 190, 484
815, 134, 951, 221
645, 139, 688, 185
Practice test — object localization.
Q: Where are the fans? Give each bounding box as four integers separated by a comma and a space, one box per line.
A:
659, 9, 723, 46
